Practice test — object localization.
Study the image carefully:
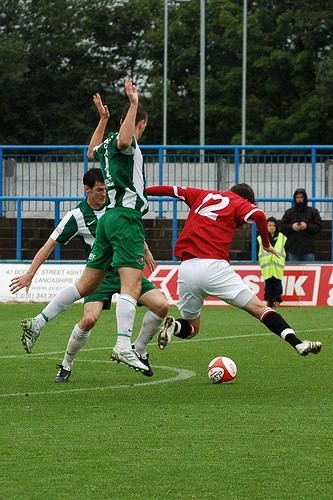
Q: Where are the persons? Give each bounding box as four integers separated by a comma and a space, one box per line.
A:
279, 189, 324, 261
145, 182, 323, 356
20, 78, 151, 374
10, 168, 170, 382
256, 216, 289, 307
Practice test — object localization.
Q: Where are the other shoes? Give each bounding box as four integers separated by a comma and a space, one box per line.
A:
273, 302, 280, 307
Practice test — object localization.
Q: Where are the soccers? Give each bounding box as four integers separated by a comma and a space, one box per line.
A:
207, 356, 238, 384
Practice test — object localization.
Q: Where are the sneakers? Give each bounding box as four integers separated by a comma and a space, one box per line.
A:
20, 317, 41, 354
54, 364, 72, 384
110, 345, 149, 373
157, 316, 176, 349
130, 345, 155, 377
296, 339, 323, 356
